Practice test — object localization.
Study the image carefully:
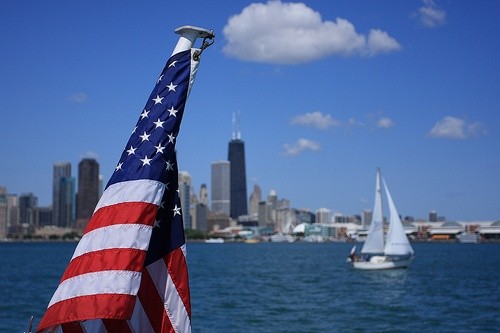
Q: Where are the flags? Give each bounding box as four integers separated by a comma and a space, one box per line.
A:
35, 47, 202, 332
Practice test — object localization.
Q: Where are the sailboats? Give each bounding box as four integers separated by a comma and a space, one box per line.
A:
352, 167, 416, 269
